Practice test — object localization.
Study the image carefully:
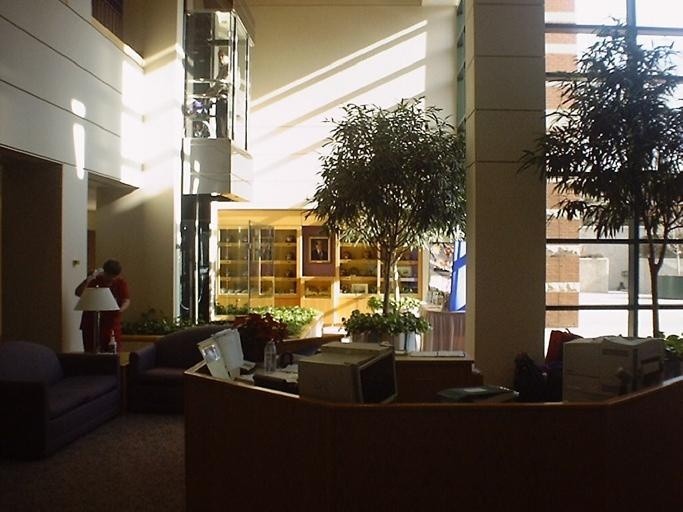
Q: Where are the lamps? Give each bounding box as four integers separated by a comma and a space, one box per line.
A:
73, 287, 121, 353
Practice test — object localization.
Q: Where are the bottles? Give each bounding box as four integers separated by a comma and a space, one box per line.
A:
264, 341, 277, 374
108, 335, 117, 353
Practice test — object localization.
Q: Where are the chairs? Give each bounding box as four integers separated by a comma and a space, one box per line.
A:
126, 324, 268, 415
0, 339, 122, 461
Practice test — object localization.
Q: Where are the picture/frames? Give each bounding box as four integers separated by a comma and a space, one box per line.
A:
309, 236, 330, 263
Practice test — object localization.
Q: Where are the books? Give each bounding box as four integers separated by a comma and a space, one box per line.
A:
438, 382, 520, 406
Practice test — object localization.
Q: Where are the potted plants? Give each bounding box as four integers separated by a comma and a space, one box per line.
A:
344, 297, 433, 351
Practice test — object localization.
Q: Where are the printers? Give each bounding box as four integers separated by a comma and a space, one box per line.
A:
560, 337, 666, 404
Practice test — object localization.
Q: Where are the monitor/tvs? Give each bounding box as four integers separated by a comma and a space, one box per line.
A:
359, 350, 400, 404
295, 340, 391, 403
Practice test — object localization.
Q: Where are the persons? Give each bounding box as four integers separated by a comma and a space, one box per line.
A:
311, 240, 327, 260
72, 259, 130, 352
202, 47, 230, 141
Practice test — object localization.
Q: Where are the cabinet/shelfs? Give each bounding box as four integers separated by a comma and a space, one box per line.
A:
215, 210, 423, 333
392, 350, 475, 402
